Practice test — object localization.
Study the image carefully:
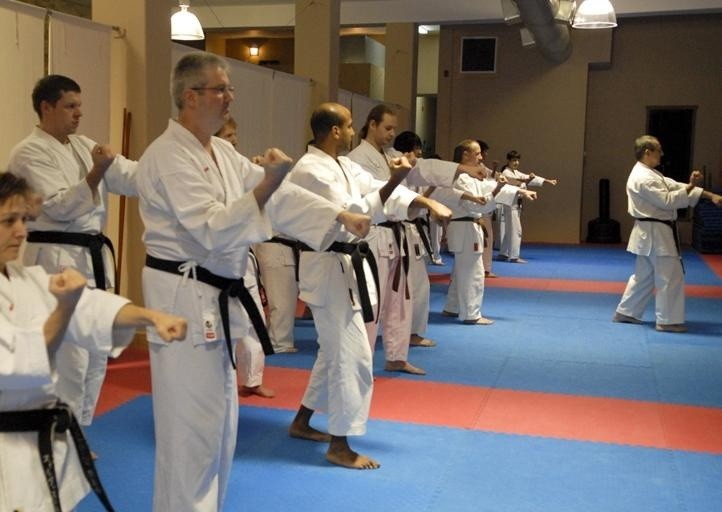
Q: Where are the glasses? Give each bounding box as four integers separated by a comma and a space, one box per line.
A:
190, 85, 236, 92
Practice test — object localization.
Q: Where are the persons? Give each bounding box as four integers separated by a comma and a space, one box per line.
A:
0, 171, 188, 512
611, 135, 722, 332
344, 104, 560, 376
211, 117, 277, 399
288, 101, 453, 470
0, 75, 139, 295
135, 50, 373, 512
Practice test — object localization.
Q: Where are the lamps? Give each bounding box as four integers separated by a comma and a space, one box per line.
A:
171, 1, 205, 41
572, 0, 619, 32
